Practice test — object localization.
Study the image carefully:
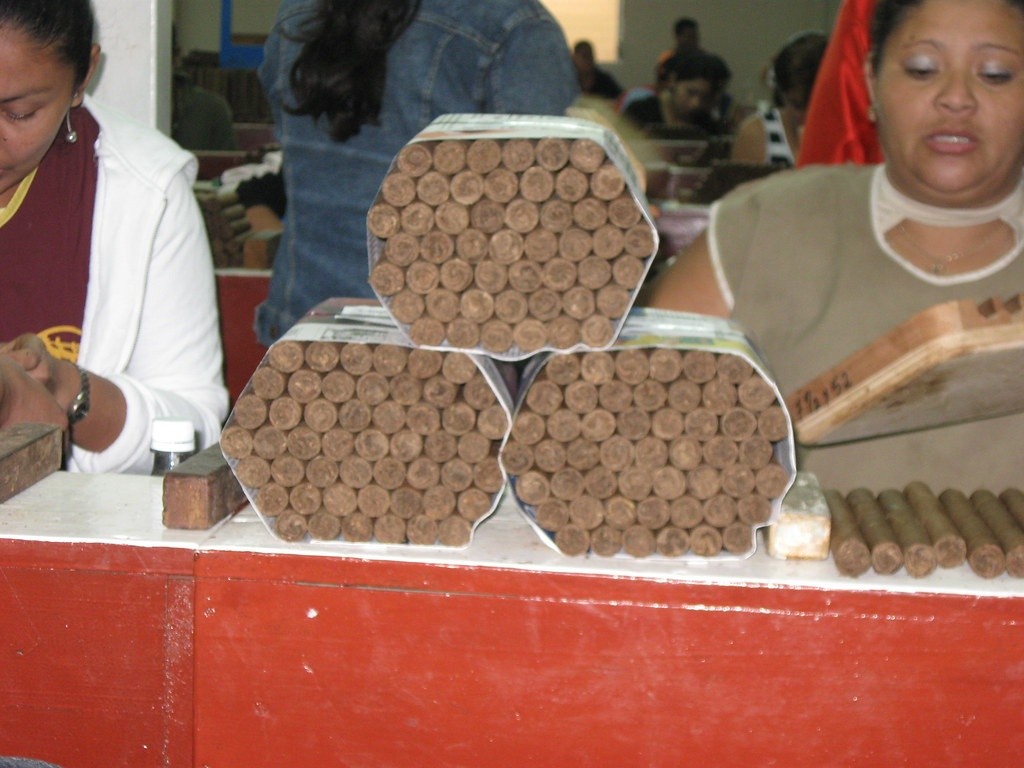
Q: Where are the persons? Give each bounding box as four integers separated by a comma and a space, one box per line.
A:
0, 1, 230, 475
572, 16, 831, 201
636, 1, 1024, 400
252, 0, 582, 368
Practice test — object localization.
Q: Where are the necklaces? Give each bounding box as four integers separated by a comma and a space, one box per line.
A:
898, 225, 985, 275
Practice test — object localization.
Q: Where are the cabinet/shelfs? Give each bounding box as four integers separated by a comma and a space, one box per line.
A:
0, 469, 1024, 768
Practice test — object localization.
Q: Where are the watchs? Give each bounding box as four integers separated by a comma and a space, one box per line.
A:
66, 364, 89, 425
